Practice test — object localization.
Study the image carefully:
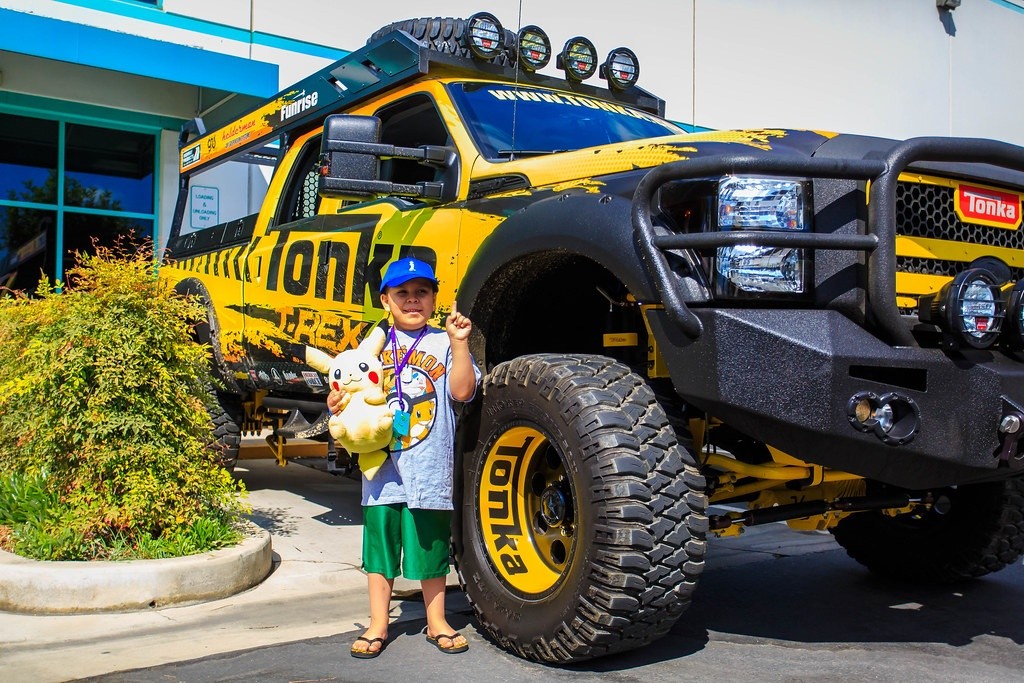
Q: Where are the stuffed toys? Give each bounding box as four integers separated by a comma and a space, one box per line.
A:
289, 319, 393, 481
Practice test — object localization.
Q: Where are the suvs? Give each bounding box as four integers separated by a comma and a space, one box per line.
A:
157, 8, 1024, 669
0, 228, 81, 297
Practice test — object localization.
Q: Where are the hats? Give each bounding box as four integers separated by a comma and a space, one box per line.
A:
379, 256, 439, 294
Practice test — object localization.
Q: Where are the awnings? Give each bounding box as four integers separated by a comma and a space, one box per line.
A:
0, 8, 279, 133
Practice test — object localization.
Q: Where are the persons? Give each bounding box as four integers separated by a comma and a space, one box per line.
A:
327, 258, 481, 660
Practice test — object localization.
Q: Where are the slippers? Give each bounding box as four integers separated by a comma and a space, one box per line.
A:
350, 636, 387, 660
425, 632, 469, 654
